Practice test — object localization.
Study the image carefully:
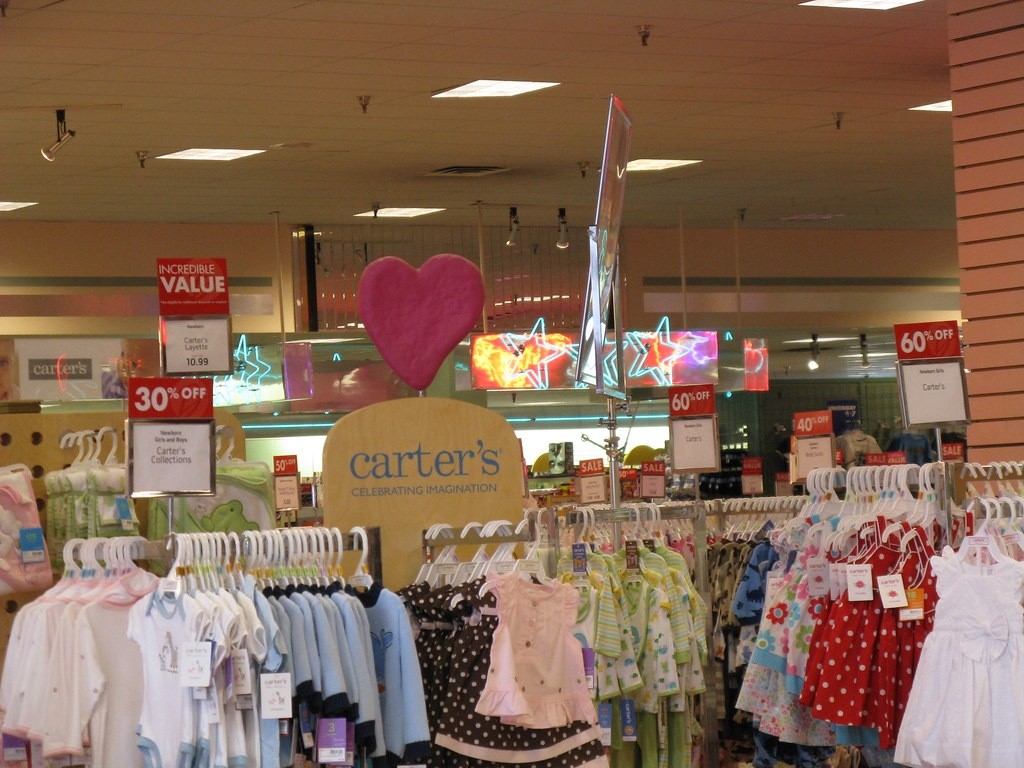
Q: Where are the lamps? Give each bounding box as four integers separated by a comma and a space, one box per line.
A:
506, 207, 520, 247
557, 208, 569, 249
42, 110, 76, 161
859, 334, 872, 370
809, 334, 821, 371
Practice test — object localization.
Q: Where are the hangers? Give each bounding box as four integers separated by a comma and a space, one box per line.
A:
62, 526, 376, 590
413, 460, 1024, 624
0, 425, 269, 478
